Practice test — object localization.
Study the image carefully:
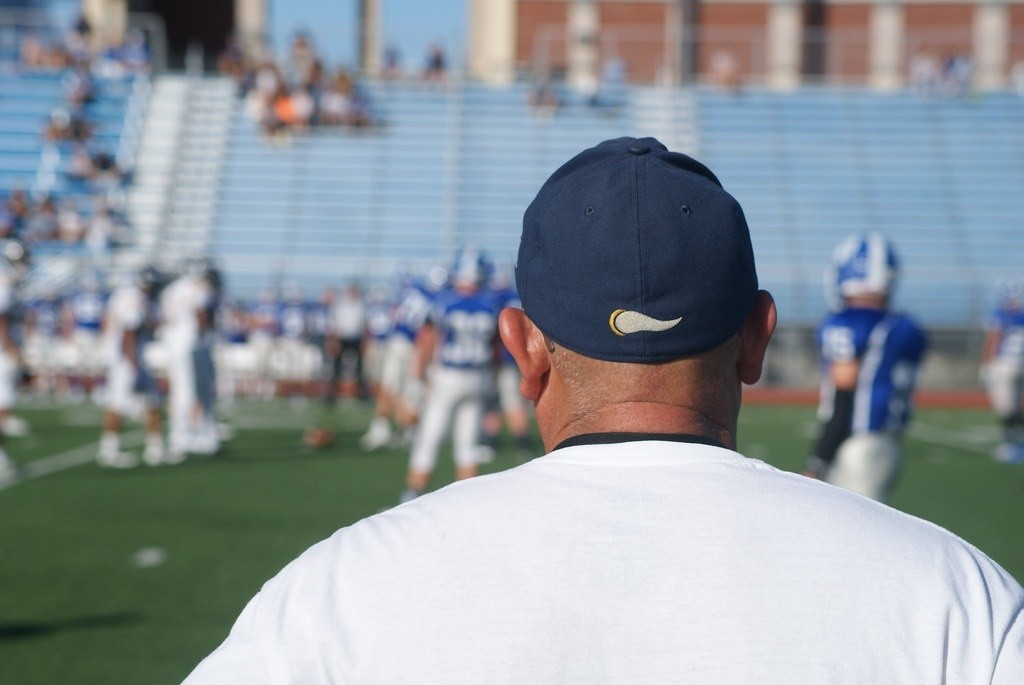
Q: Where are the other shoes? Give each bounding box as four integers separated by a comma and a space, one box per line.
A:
94, 451, 138, 469
475, 445, 498, 463
143, 447, 184, 465
167, 420, 234, 455
359, 424, 394, 450
993, 442, 1024, 462
0, 418, 29, 438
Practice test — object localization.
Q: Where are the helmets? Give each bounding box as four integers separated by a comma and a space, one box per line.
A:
837, 234, 899, 296
448, 247, 496, 283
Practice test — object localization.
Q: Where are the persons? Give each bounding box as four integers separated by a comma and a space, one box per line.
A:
804, 230, 937, 509
983, 291, 1024, 461
0, 0, 634, 467
179, 137, 1024, 685
400, 248, 516, 504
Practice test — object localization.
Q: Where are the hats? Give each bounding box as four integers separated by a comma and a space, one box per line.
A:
514, 137, 759, 364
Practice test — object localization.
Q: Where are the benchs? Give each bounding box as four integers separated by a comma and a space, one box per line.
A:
1, 64, 1023, 342
